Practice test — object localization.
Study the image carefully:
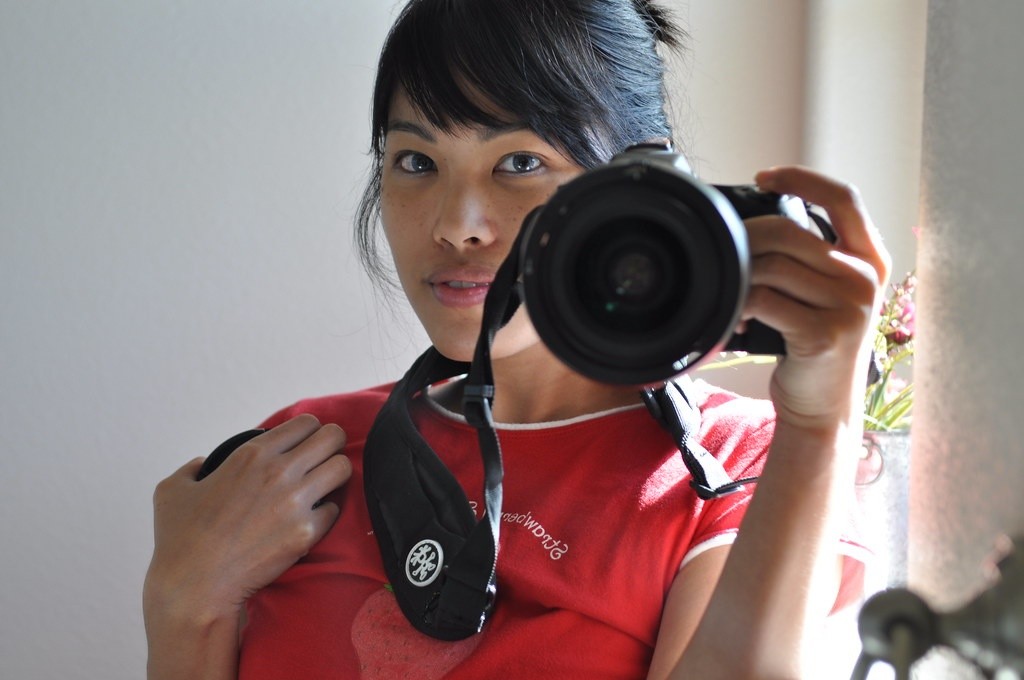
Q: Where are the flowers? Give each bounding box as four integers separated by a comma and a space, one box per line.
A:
866, 271, 917, 434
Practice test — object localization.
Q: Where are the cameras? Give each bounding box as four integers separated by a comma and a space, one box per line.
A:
518, 138, 850, 393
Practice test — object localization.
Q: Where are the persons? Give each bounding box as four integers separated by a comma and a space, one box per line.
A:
143, 0, 892, 680
